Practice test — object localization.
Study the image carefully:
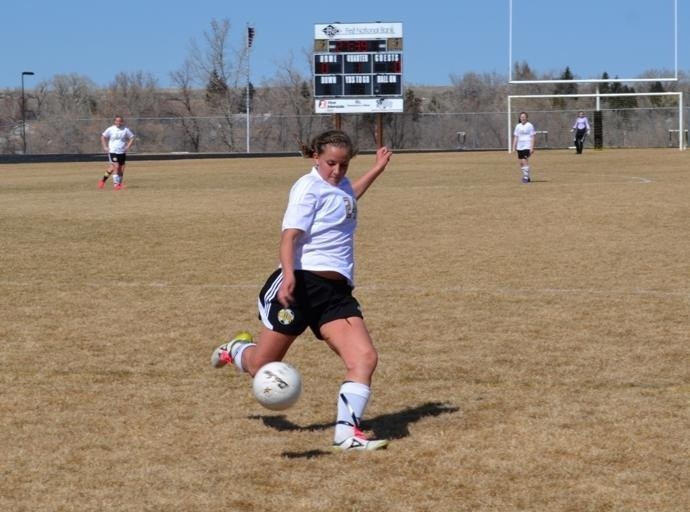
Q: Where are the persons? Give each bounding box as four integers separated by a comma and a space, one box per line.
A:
570, 111, 591, 154
512, 112, 536, 183
210, 131, 393, 454
98, 115, 135, 190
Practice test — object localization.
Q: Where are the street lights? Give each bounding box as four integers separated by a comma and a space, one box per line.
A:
20, 71, 36, 155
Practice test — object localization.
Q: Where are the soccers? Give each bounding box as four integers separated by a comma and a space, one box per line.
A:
254, 362, 302, 408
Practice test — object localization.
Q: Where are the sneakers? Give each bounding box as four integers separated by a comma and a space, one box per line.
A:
331, 424, 389, 450
209, 330, 253, 369
523, 175, 529, 183
